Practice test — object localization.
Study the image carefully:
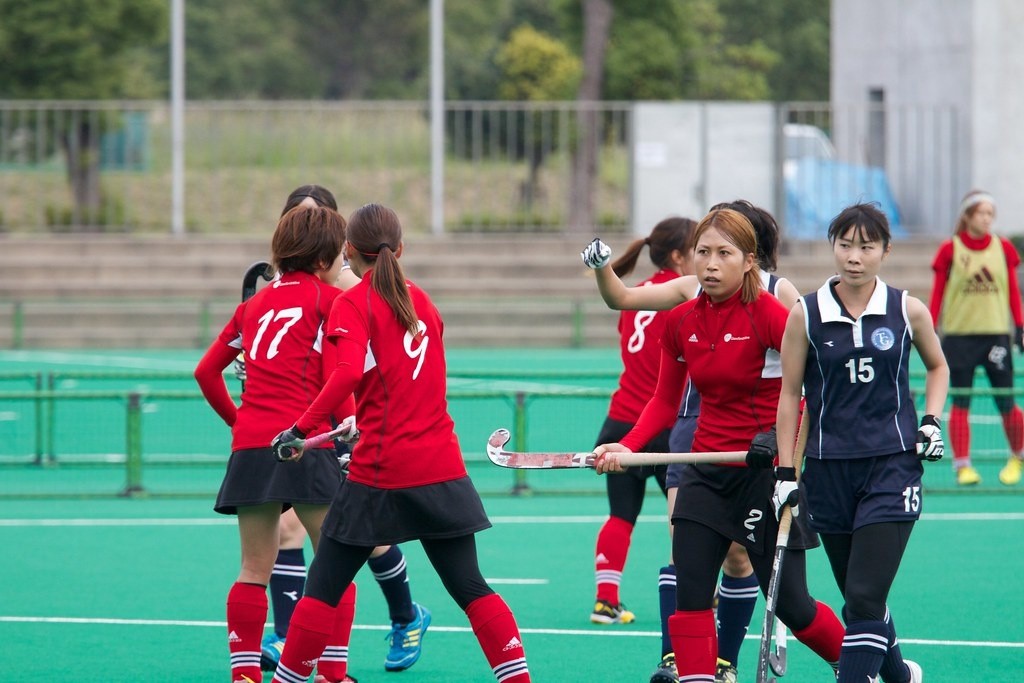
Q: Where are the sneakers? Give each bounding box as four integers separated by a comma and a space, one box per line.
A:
591, 600, 635, 624
650, 656, 679, 683
384, 600, 431, 671
260, 635, 286, 668
714, 657, 738, 683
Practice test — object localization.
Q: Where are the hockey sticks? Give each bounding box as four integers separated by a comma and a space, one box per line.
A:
769, 617, 788, 677
241, 261, 274, 393
486, 427, 751, 470
277, 423, 352, 462
754, 399, 810, 683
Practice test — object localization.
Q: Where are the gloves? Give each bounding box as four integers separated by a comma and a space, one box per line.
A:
335, 416, 359, 444
270, 423, 305, 461
745, 426, 778, 470
914, 415, 943, 462
1016, 329, 1024, 352
581, 237, 611, 270
770, 467, 800, 522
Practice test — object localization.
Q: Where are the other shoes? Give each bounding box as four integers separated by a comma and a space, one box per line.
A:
957, 465, 980, 486
998, 452, 1023, 484
314, 673, 357, 683
900, 659, 921, 683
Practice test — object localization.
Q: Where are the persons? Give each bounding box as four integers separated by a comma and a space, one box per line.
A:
772, 201, 950, 683
929, 191, 1024, 485
592, 208, 880, 683
590, 217, 718, 625
61, 95, 105, 227
271, 203, 531, 682
194, 207, 357, 683
234, 186, 431, 671
581, 201, 801, 683
